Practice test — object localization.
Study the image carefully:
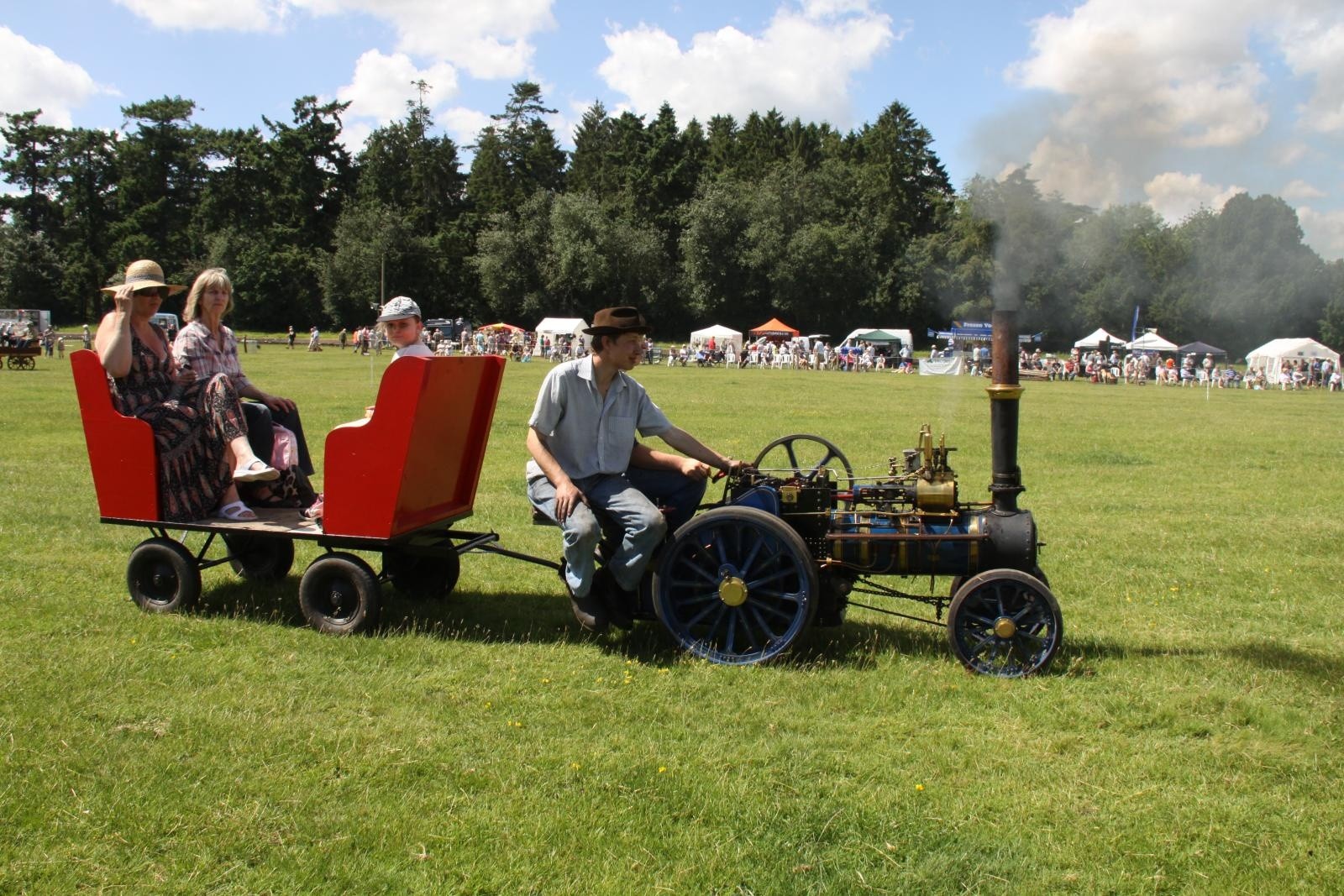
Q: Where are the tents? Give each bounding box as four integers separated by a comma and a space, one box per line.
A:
532, 317, 591, 356
854, 330, 902, 368
1074, 328, 1228, 380
748, 318, 799, 343
690, 325, 743, 363
1243, 337, 1341, 384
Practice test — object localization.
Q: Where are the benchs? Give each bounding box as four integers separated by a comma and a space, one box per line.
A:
69, 348, 160, 521
324, 355, 506, 539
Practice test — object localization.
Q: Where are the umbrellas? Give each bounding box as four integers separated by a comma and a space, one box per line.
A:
477, 323, 525, 333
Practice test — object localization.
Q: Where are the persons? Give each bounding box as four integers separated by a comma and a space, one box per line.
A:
94, 259, 280, 521
285, 326, 296, 349
1020, 347, 1342, 392
931, 344, 990, 377
638, 334, 915, 375
167, 324, 177, 346
80, 324, 91, 350
298, 296, 436, 521
339, 326, 398, 357
172, 268, 324, 508
307, 326, 323, 352
421, 327, 585, 363
525, 307, 753, 633
0, 322, 65, 360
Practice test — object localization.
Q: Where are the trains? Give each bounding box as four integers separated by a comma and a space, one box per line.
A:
70, 308, 1066, 680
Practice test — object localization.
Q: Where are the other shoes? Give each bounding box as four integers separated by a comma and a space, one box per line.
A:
593, 568, 633, 631
565, 581, 609, 632
298, 494, 324, 522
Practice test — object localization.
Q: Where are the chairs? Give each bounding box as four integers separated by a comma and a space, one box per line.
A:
1111, 368, 1119, 379
1155, 370, 1256, 387
667, 350, 828, 371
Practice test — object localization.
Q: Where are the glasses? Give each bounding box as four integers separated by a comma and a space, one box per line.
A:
130, 286, 169, 300
387, 323, 418, 329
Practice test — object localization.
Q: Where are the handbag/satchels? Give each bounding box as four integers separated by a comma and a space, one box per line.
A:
265, 421, 299, 502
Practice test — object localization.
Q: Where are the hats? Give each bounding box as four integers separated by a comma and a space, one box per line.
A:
100, 259, 188, 296
375, 296, 421, 321
581, 307, 653, 335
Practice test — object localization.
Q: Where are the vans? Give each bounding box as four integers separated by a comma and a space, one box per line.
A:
149, 313, 179, 342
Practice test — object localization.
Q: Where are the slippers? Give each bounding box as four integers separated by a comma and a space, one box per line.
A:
214, 501, 259, 521
250, 488, 283, 508
232, 459, 280, 482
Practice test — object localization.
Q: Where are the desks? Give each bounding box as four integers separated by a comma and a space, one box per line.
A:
919, 357, 963, 376
